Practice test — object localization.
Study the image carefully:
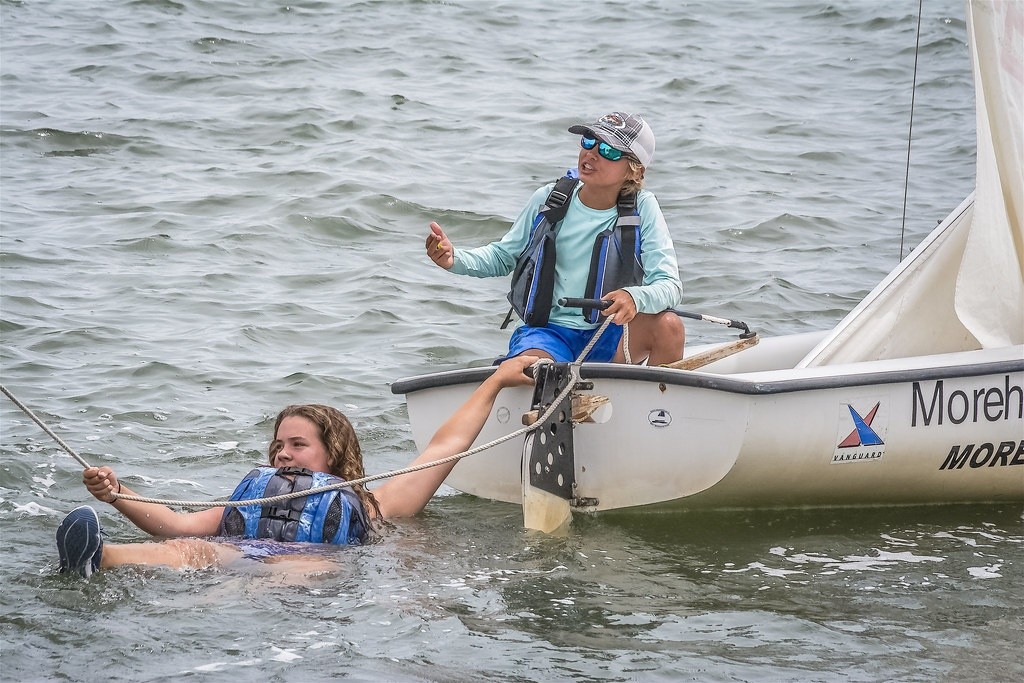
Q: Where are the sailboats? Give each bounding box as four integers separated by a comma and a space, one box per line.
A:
399, 1, 1022, 515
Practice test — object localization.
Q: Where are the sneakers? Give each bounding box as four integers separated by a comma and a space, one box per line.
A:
56, 505, 103, 579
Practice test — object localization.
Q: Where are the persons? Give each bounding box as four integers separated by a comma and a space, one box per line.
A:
57, 355, 540, 570
425, 112, 685, 366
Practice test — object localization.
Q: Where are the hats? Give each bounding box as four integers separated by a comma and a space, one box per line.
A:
569, 111, 655, 170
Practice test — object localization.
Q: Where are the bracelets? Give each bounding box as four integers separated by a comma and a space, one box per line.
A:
106, 481, 121, 504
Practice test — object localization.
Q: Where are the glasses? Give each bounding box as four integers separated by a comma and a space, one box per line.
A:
580, 133, 629, 161
